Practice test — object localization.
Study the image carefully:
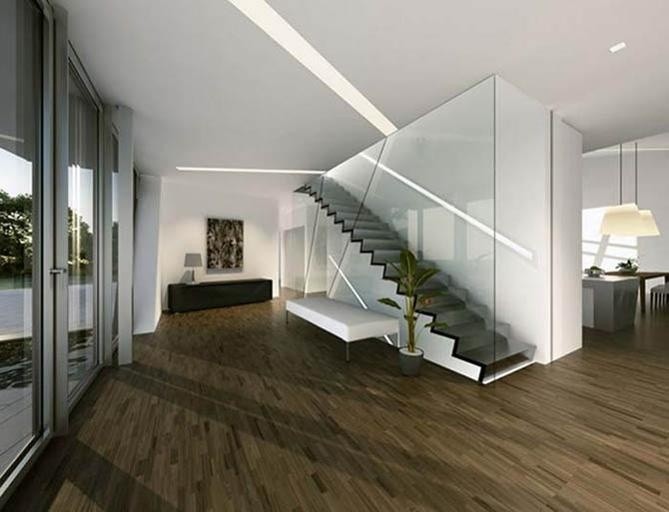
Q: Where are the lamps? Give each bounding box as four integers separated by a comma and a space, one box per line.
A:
598, 142, 661, 238
184, 253, 202, 286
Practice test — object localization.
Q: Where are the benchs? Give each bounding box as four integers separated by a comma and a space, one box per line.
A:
285, 295, 400, 363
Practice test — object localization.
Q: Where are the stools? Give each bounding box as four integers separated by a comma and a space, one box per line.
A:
650, 284, 669, 312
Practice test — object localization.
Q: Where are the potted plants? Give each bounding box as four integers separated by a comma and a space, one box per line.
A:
376, 247, 449, 378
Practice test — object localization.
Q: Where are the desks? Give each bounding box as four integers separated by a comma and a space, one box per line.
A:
605, 271, 669, 313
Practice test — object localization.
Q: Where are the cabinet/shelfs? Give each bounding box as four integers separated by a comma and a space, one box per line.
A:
168, 278, 272, 313
